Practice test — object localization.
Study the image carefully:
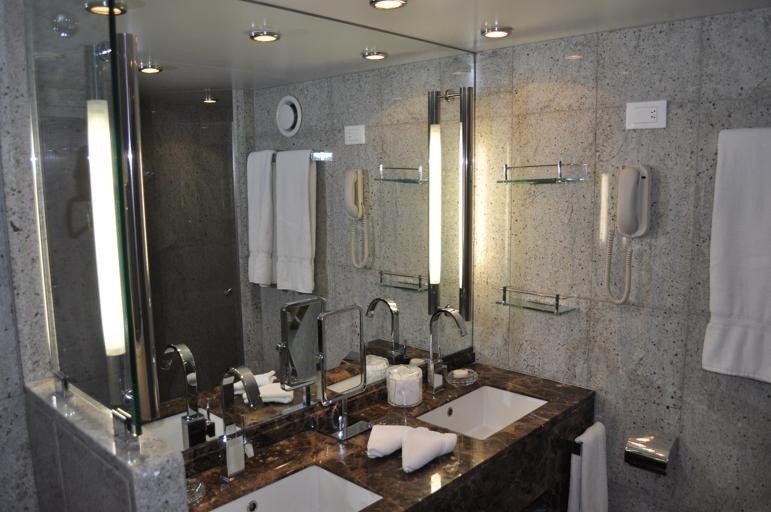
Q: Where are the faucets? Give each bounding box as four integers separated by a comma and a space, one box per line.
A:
162, 341, 200, 417
427, 307, 468, 361
362, 296, 401, 351
220, 364, 264, 436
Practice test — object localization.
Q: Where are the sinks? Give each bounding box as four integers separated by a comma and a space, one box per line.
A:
415, 381, 550, 442
202, 462, 383, 512
325, 373, 361, 394
139, 406, 241, 453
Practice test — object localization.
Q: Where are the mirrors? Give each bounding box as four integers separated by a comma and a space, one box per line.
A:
25, 1, 476, 438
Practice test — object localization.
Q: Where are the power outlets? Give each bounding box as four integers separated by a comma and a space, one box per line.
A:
624, 101, 669, 128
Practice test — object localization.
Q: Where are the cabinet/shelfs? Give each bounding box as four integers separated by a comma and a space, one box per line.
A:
373, 160, 429, 293
497, 156, 589, 317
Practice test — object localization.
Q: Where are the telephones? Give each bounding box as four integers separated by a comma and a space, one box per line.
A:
613, 161, 655, 241
341, 166, 366, 224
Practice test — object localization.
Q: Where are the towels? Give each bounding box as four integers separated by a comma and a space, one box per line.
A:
278, 149, 320, 294
241, 381, 296, 405
362, 424, 415, 461
245, 150, 278, 288
567, 421, 614, 510
227, 370, 278, 395
400, 425, 457, 474
700, 120, 770, 384
